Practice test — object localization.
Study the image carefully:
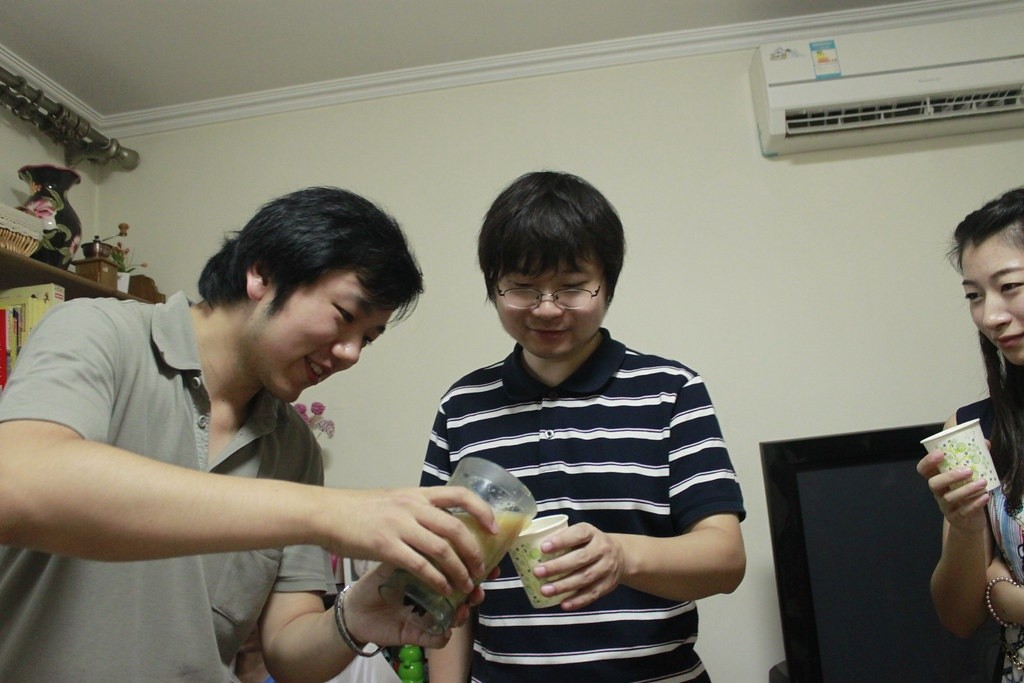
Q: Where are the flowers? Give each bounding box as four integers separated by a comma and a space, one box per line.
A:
109, 242, 135, 273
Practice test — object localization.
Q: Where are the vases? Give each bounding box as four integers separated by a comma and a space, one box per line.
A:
18, 163, 82, 270
118, 272, 130, 294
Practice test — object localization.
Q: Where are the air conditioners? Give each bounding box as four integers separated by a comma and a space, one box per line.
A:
750, 1, 1023, 158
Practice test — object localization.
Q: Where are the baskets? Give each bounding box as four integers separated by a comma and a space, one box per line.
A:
0, 205, 42, 257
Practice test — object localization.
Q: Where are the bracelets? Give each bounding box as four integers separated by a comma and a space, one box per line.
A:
986, 576, 1021, 628
334, 578, 389, 658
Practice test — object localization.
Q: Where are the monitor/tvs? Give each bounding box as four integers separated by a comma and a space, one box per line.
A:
760, 420, 1008, 683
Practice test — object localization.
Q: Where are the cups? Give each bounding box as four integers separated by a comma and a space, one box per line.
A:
378, 456, 537, 635
507, 514, 577, 609
920, 418, 1001, 501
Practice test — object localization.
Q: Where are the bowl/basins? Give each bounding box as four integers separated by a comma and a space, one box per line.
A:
80, 235, 112, 259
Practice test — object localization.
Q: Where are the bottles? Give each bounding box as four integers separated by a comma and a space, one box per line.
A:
17, 163, 83, 271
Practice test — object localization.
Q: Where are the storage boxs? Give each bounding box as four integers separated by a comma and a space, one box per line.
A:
0, 282, 64, 372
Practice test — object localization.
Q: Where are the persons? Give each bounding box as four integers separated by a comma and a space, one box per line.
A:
915, 186, 1024, 683
1, 185, 500, 683
419, 171, 747, 683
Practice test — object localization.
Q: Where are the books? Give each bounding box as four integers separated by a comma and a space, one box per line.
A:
0, 283, 67, 395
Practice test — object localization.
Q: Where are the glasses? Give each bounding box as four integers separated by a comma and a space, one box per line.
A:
495, 269, 603, 310
